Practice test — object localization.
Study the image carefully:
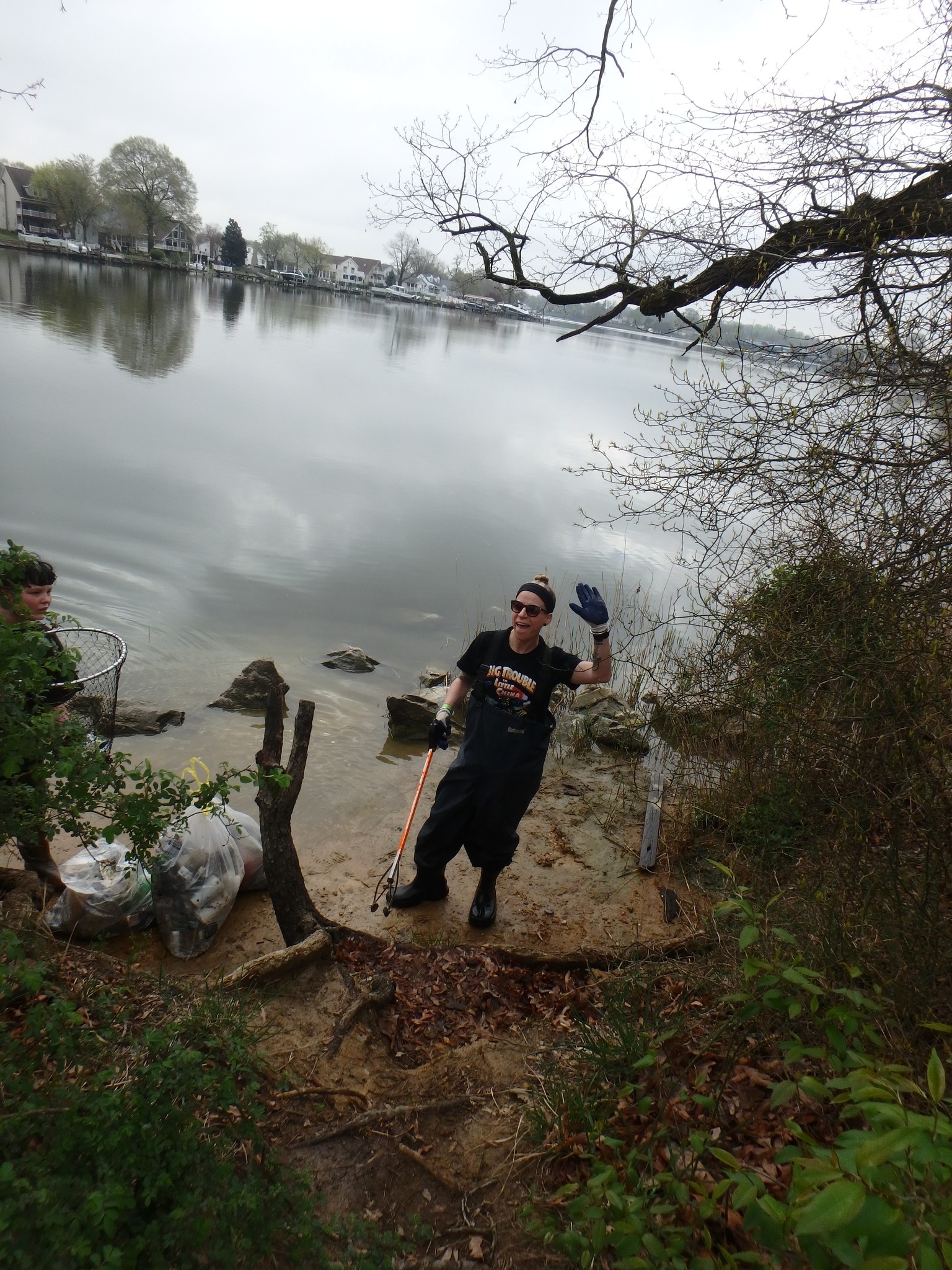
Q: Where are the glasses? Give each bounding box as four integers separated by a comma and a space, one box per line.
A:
511, 599, 550, 617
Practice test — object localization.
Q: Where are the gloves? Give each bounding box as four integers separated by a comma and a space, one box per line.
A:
568, 584, 610, 640
429, 708, 453, 750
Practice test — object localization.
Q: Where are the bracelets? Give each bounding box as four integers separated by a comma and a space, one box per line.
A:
593, 630, 609, 640
442, 704, 452, 712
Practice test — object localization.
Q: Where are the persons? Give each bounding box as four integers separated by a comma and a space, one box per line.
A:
385, 574, 611, 928
0, 549, 88, 897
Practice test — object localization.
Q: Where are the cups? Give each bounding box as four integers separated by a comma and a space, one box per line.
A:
190, 874, 224, 910
193, 888, 234, 924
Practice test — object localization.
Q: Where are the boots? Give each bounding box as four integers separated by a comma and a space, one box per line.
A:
468, 866, 503, 926
386, 864, 448, 907
